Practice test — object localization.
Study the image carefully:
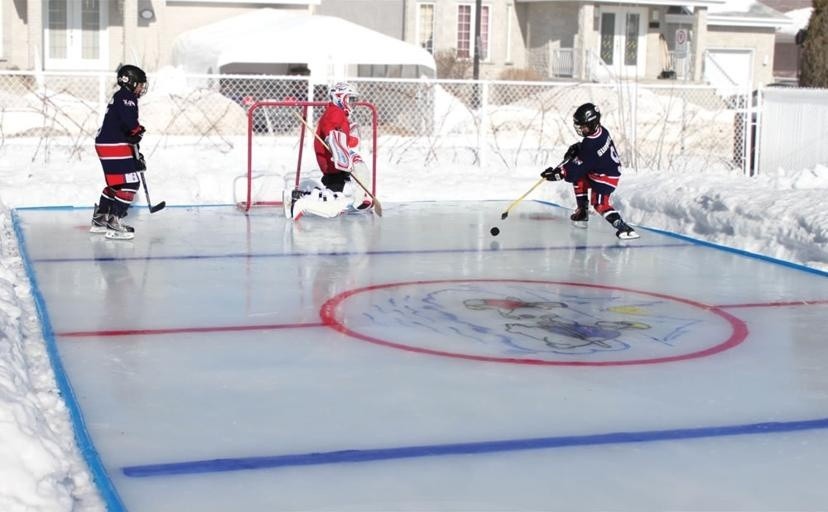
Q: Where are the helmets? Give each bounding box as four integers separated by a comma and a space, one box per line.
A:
329, 83, 360, 120
573, 103, 600, 137
118, 65, 149, 98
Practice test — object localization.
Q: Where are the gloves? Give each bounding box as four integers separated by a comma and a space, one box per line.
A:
133, 153, 146, 171
541, 167, 565, 181
564, 142, 581, 161
126, 123, 145, 144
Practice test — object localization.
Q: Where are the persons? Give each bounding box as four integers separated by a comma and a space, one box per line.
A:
89, 64, 146, 243
539, 100, 642, 239
282, 79, 373, 223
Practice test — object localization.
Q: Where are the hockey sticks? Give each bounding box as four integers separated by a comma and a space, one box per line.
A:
134, 145, 165, 213
491, 158, 570, 236
290, 107, 381, 216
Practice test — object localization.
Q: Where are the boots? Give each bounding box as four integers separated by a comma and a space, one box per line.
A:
107, 213, 134, 232
616, 219, 633, 237
571, 204, 588, 221
291, 191, 305, 217
92, 202, 109, 227
